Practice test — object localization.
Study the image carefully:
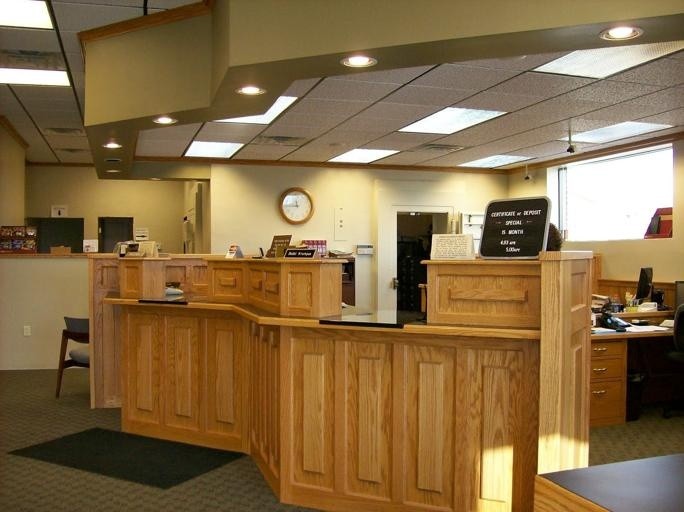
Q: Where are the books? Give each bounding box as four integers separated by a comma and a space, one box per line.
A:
625, 326, 668, 332
330, 250, 354, 258
592, 327, 616, 334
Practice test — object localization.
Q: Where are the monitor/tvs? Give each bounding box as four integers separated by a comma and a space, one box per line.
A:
675, 280, 684, 312
636, 268, 652, 299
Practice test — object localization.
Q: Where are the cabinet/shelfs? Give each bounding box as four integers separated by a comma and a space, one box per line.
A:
342, 256, 355, 307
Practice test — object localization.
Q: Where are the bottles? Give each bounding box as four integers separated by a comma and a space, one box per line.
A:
591, 308, 597, 326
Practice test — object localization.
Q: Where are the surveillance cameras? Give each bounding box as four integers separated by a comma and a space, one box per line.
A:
567, 144, 576, 153
524, 175, 531, 181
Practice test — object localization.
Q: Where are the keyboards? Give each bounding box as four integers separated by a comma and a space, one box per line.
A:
660, 319, 674, 328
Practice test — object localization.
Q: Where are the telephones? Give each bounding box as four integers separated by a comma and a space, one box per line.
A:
600, 312, 631, 328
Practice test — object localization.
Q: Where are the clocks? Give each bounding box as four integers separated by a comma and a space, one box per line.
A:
278, 186, 315, 226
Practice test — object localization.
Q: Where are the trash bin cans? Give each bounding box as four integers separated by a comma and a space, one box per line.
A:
627, 372, 645, 421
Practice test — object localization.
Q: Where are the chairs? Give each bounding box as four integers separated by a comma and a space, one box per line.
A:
664, 304, 684, 419
55, 316, 90, 399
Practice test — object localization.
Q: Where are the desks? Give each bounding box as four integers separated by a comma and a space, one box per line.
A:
591, 319, 673, 429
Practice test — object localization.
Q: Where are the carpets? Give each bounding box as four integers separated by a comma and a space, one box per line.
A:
7, 426, 245, 490
